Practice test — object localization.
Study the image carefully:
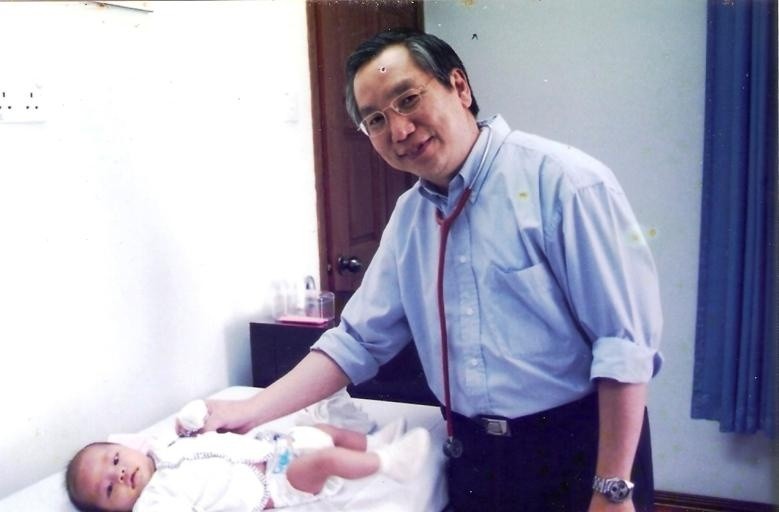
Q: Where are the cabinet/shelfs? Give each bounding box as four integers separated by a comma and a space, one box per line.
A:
247, 288, 438, 402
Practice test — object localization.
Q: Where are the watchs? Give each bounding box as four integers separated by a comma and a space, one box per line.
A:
592, 475, 635, 504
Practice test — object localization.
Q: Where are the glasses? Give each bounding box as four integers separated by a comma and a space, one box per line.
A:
355, 74, 435, 137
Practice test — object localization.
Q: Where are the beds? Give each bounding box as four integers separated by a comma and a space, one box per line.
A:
1, 385, 453, 510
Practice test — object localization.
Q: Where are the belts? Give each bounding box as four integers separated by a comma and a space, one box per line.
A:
440, 391, 599, 439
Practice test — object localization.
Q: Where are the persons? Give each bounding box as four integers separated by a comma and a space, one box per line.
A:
66, 399, 431, 512
176, 28, 665, 512
